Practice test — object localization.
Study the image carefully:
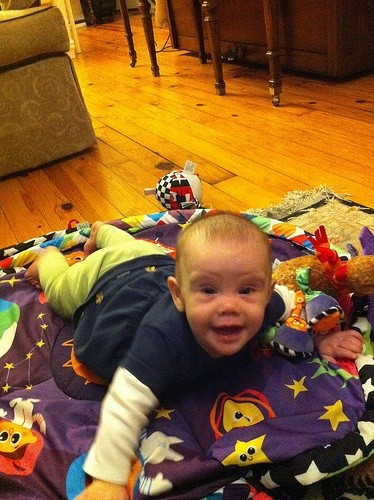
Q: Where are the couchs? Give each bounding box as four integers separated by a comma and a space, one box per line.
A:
0, 1, 95, 178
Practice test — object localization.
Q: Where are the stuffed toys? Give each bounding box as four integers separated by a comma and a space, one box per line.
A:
264, 295, 314, 361
271, 225, 374, 322
291, 266, 344, 337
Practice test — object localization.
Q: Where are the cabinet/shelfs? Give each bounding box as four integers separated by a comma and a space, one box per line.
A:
161, 0, 371, 79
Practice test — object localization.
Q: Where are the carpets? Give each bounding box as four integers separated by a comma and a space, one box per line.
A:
243, 184, 372, 256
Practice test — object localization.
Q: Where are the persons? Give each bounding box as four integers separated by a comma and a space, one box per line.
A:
24, 217, 364, 499
